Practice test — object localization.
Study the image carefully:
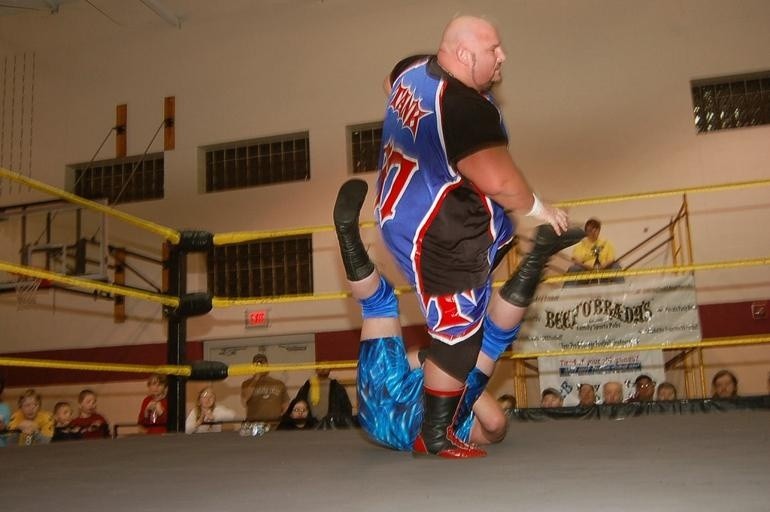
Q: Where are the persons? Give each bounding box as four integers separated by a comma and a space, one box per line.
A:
497, 393, 517, 409
0, 379, 112, 448
184, 387, 236, 436
575, 374, 677, 406
276, 397, 320, 430
711, 369, 740, 398
561, 217, 625, 289
372, 15, 571, 460
297, 368, 353, 419
539, 387, 564, 408
240, 353, 291, 421
332, 178, 586, 452
137, 375, 168, 434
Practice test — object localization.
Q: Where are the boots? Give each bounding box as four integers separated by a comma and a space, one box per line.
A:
499, 225, 586, 308
413, 386, 488, 459
333, 178, 375, 282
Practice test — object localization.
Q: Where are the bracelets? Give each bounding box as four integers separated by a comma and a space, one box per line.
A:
524, 193, 543, 219
